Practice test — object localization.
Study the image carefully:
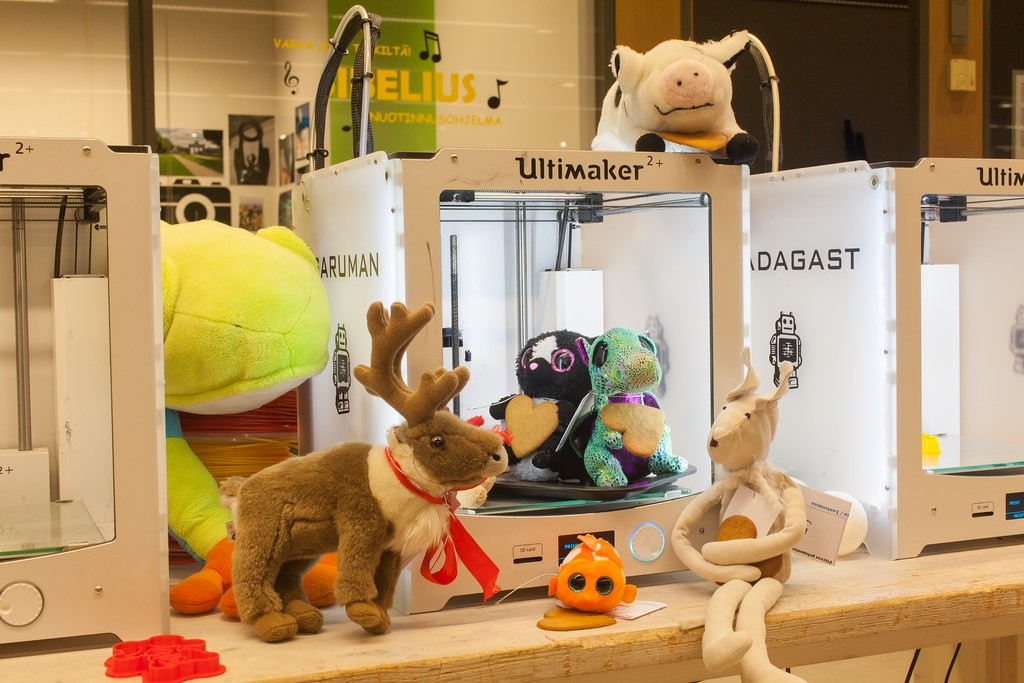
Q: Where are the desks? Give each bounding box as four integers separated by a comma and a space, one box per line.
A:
1, 532, 1024, 683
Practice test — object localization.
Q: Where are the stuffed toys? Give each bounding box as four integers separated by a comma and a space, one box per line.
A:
217, 300, 510, 643
161, 217, 332, 564
170, 532, 339, 618
591, 29, 760, 166
671, 350, 810, 683
547, 534, 637, 614
570, 327, 688, 491
488, 326, 601, 483
